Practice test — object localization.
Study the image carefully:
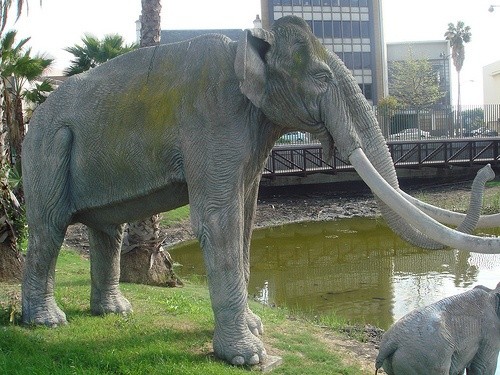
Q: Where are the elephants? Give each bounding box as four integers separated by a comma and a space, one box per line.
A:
17, 15, 500, 367
376, 281, 500, 375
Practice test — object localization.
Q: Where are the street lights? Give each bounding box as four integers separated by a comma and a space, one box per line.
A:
440, 51, 450, 136
2, 74, 19, 172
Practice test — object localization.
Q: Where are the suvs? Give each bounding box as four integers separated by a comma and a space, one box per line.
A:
471, 127, 488, 136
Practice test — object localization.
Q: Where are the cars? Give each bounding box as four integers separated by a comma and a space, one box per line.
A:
389, 128, 430, 140
481, 129, 498, 137
281, 130, 306, 143
